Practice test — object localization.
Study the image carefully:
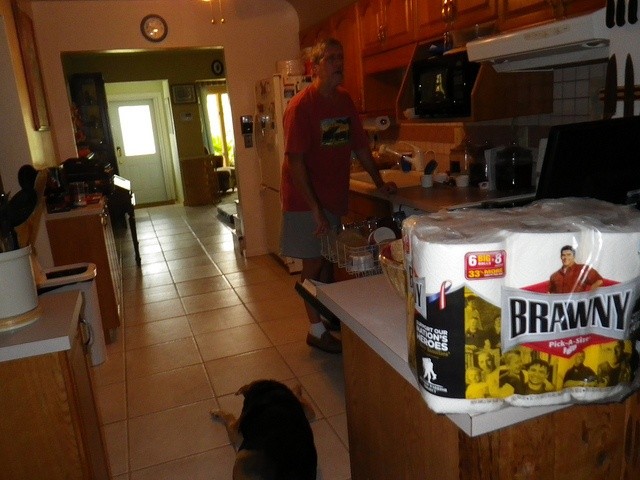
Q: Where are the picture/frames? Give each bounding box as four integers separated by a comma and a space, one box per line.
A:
170, 84, 197, 103
11, 3, 50, 132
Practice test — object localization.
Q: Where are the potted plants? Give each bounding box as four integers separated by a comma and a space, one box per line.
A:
0, 164, 40, 334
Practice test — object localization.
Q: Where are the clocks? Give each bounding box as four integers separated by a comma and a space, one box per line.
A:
141, 13, 168, 41
212, 60, 223, 75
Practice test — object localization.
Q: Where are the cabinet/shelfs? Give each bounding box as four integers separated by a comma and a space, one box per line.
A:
497, 0, 607, 35
301, 1, 409, 112
44, 195, 122, 329
411, 1, 497, 44
0, 290, 111, 480
357, 0, 411, 58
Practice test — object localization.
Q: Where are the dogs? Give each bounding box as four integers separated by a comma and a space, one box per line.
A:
209, 379, 317, 480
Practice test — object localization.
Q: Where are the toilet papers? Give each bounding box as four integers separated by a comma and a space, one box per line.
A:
401, 197, 636, 414
364, 113, 391, 133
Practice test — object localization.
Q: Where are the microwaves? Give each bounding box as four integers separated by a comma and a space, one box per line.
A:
412, 52, 478, 119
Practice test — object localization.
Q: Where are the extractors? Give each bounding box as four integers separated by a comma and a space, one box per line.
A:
466, 7, 611, 73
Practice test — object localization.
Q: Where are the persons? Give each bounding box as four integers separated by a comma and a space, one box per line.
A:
479, 349, 499, 377
546, 245, 602, 294
466, 318, 492, 347
279, 37, 400, 354
465, 293, 481, 338
489, 315, 500, 343
598, 339, 635, 387
527, 360, 554, 393
466, 367, 488, 398
564, 351, 596, 388
489, 349, 527, 396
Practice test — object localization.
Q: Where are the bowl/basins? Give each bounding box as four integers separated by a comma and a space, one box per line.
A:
368, 227, 396, 247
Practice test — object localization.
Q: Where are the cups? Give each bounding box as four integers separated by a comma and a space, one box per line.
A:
422, 175, 434, 188
455, 175, 469, 187
436, 173, 449, 184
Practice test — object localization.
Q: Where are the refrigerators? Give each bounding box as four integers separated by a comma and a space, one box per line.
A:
254, 75, 312, 274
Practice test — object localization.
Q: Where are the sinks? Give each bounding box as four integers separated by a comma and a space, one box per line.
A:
350, 169, 424, 191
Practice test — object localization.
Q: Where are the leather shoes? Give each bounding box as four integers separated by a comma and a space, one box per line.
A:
323, 319, 340, 331
307, 330, 342, 354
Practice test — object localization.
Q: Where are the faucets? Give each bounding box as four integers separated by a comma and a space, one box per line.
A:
378, 142, 424, 172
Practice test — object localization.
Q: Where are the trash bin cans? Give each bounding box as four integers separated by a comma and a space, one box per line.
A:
38, 262, 106, 367
